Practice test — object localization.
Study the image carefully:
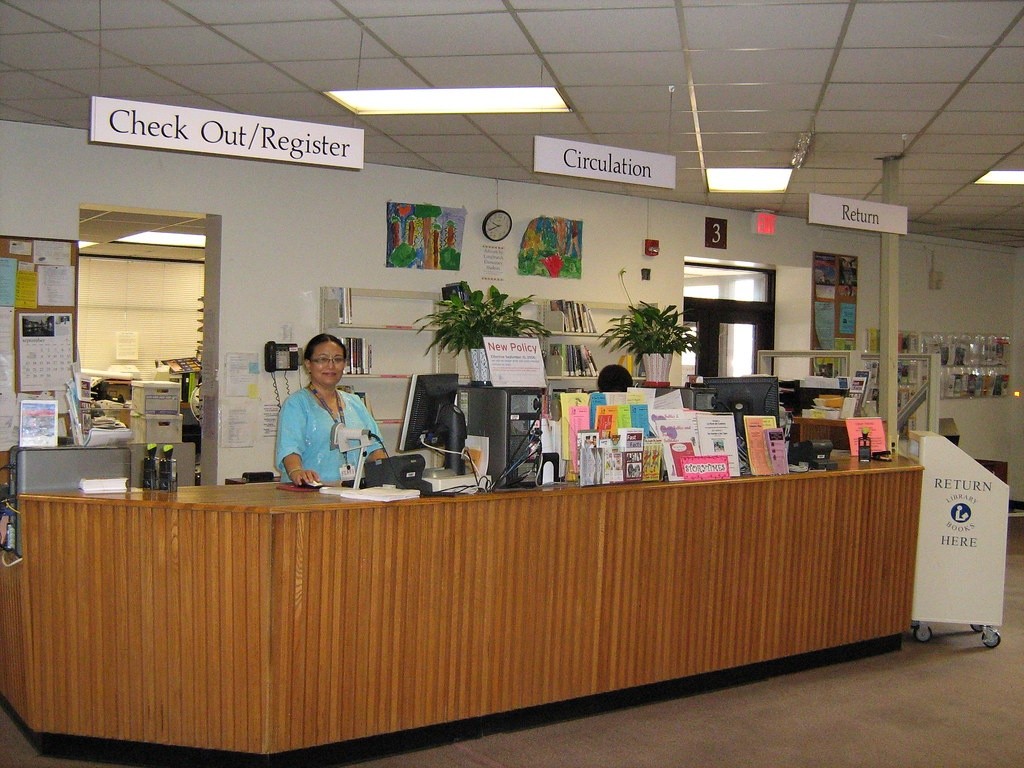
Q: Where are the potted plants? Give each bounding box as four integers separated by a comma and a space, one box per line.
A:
412, 286, 554, 388
598, 300, 703, 388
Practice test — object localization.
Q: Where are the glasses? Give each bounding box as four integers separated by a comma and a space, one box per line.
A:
311, 357, 345, 364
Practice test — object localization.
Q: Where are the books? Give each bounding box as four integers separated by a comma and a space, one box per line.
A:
465, 435, 489, 475
80, 475, 128, 491
551, 300, 598, 333
339, 288, 352, 324
338, 337, 373, 375
549, 343, 599, 377
20, 400, 58, 447
341, 486, 420, 502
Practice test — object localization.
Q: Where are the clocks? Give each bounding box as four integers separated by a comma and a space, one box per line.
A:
482, 210, 513, 242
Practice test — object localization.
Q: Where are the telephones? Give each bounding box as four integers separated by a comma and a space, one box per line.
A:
265, 340, 299, 372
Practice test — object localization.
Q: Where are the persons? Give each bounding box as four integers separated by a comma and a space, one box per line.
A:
275, 333, 387, 486
598, 365, 633, 392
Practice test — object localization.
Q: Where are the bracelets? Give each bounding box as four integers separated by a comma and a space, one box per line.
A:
289, 468, 303, 478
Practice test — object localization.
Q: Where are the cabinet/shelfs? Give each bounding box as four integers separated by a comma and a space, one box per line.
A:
319, 286, 659, 424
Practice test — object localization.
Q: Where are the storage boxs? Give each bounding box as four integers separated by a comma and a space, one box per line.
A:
130, 380, 183, 444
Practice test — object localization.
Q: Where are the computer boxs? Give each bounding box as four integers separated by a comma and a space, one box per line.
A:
655, 386, 718, 413
459, 386, 542, 490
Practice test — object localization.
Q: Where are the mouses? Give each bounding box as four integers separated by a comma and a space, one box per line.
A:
298, 479, 323, 489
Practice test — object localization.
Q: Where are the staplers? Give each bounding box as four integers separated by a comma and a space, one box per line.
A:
872, 451, 892, 462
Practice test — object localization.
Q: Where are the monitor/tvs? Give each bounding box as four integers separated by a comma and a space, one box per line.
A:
398, 373, 473, 479
689, 376, 780, 461
